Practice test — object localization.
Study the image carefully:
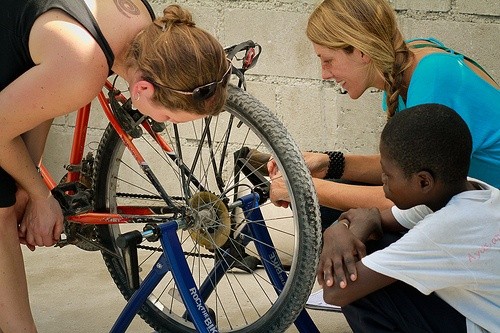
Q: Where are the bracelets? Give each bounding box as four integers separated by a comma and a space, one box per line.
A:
325, 150, 347, 181
324, 222, 350, 234
36, 167, 41, 172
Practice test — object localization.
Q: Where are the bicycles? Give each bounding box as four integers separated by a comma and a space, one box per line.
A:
13, 60, 323, 333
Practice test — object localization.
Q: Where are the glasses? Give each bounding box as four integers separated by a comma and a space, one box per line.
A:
142, 58, 232, 101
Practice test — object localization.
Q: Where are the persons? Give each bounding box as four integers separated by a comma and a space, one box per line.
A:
1, 1, 231, 333
316, 103, 500, 333
268, 0, 499, 236
